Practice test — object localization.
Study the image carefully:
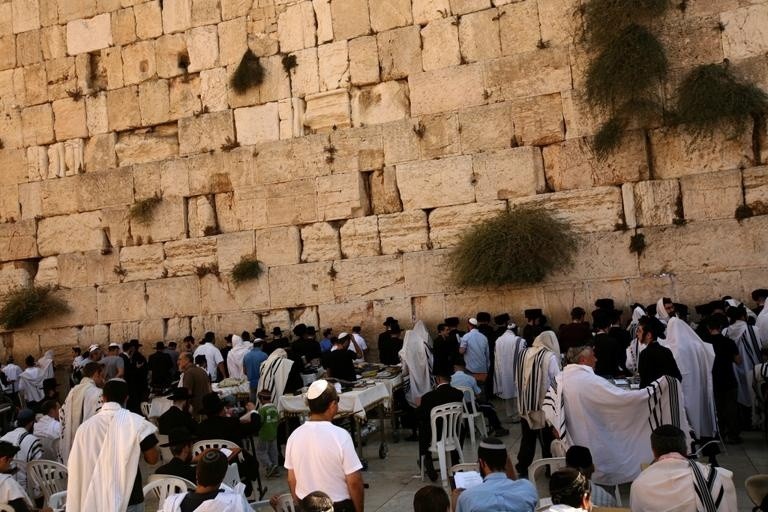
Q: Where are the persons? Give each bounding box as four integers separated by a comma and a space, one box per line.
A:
1, 287, 768, 512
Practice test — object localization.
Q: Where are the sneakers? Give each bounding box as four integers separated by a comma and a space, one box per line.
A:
488, 428, 509, 436
265, 464, 280, 478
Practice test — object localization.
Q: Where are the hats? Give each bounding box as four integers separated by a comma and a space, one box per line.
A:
468, 318, 477, 325
153, 342, 166, 349
549, 467, 584, 496
159, 430, 189, 447
307, 379, 328, 399
307, 326, 318, 336
195, 392, 228, 415
225, 327, 283, 340
258, 389, 272, 396
167, 388, 193, 400
640, 315, 667, 338
384, 317, 403, 333
199, 449, 225, 469
88, 340, 143, 352
338, 333, 347, 339
40, 378, 60, 390
0, 441, 20, 458
